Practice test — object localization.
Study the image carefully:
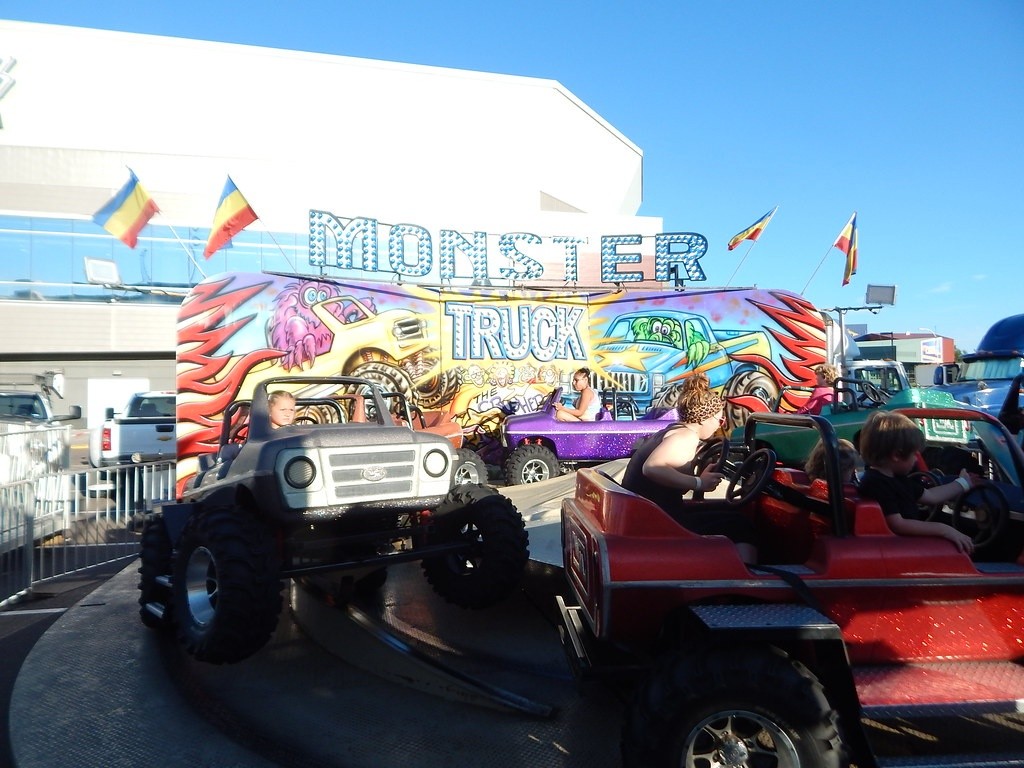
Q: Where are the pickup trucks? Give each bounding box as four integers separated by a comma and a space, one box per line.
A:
98, 389, 177, 482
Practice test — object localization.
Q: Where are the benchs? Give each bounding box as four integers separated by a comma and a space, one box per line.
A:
574, 468, 744, 581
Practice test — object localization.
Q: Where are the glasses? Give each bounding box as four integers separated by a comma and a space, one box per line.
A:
713, 414, 726, 426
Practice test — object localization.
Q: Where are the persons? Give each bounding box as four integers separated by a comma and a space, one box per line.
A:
551, 366, 601, 422
620, 372, 757, 567
267, 390, 296, 430
805, 409, 1024, 574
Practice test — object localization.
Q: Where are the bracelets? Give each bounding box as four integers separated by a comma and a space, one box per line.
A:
954, 478, 969, 493
695, 477, 701, 491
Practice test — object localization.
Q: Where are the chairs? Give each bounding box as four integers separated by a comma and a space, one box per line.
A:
140, 403, 161, 417
806, 475, 894, 541
752, 462, 815, 548
15, 403, 36, 417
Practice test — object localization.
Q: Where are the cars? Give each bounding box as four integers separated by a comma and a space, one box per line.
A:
496, 314, 1024, 568
553, 407, 1023, 768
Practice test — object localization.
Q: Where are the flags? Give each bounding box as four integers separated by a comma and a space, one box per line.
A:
202, 175, 260, 260
92, 170, 159, 248
833, 211, 859, 288
728, 206, 777, 251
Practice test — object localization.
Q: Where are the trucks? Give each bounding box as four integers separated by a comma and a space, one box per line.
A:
1, 369, 81, 484
138, 373, 532, 666
